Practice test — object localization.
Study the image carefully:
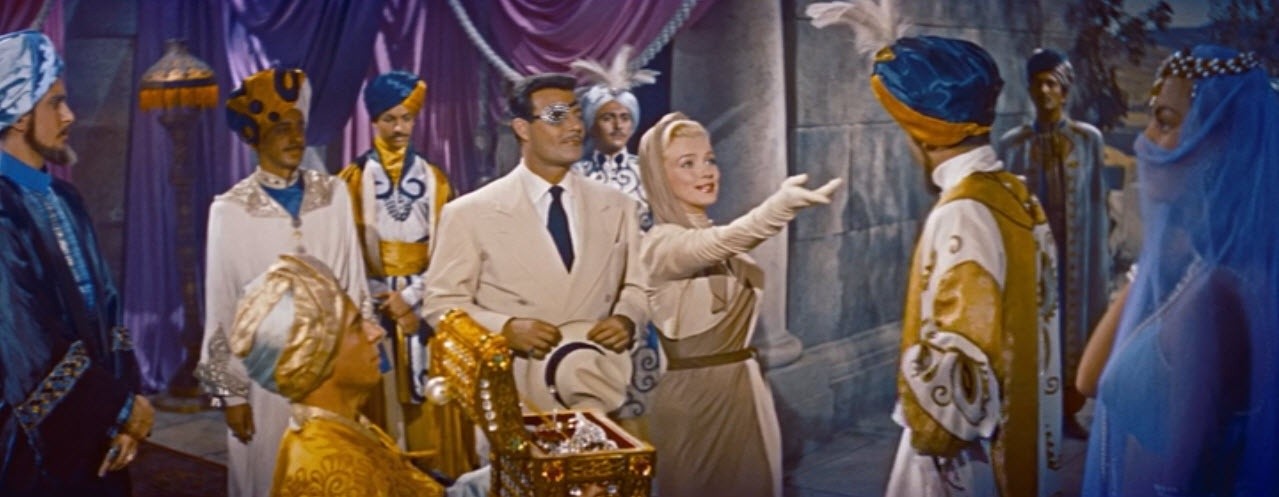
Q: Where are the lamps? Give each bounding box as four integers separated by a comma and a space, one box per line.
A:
137, 39, 221, 398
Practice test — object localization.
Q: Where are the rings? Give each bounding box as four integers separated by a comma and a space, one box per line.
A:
108, 447, 117, 457
127, 454, 134, 461
527, 347, 535, 355
232, 432, 237, 436
146, 432, 151, 438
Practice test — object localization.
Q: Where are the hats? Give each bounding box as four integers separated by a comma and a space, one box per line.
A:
227, 252, 343, 400
805, 0, 1001, 144
226, 70, 311, 148
365, 72, 426, 122
569, 43, 661, 134
0, 30, 65, 131
525, 319, 633, 416
1025, 47, 1075, 92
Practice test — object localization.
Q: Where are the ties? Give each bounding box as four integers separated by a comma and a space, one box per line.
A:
547, 186, 574, 274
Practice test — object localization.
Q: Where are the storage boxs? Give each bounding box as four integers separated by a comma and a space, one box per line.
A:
429, 306, 656, 497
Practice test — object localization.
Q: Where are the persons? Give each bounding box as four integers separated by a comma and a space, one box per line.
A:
338, 71, 480, 479
227, 253, 489, 497
999, 48, 1108, 441
0, 29, 154, 497
570, 81, 654, 232
191, 67, 392, 497
869, 34, 1061, 497
638, 112, 842, 497
422, 71, 651, 464
1076, 42, 1279, 497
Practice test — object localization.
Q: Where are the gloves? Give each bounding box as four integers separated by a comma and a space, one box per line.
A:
772, 173, 842, 218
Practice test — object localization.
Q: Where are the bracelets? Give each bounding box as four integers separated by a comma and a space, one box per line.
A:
1126, 264, 1139, 282
